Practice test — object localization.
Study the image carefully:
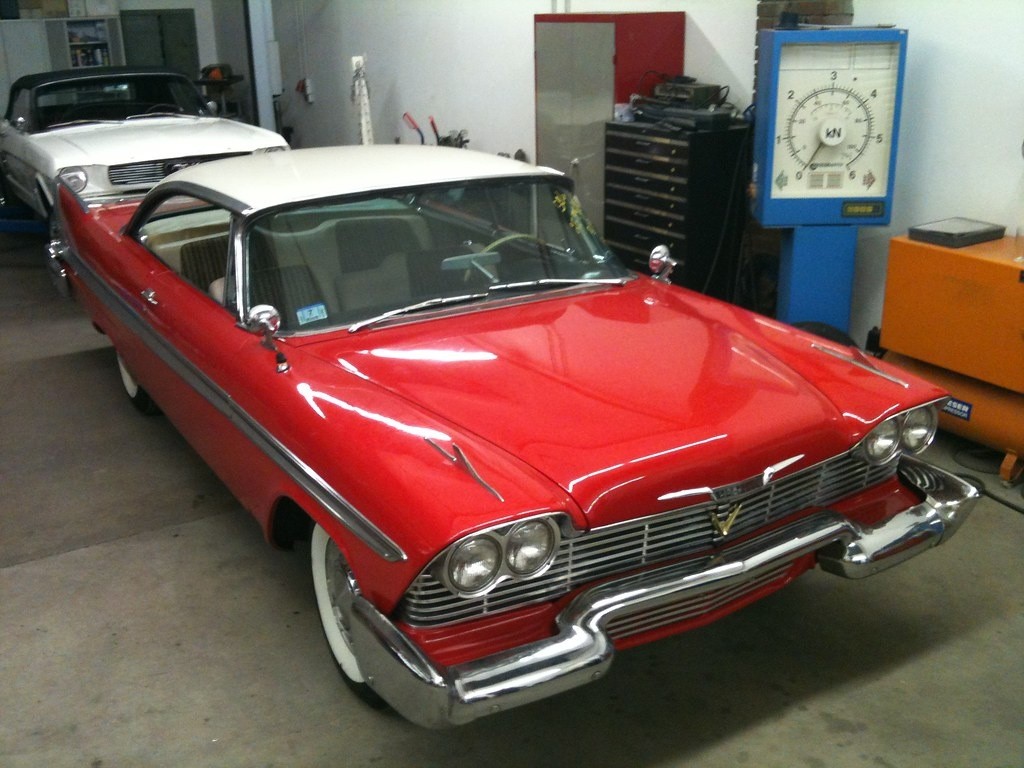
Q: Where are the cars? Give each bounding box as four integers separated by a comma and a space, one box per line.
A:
0, 64, 294, 220
47, 141, 986, 729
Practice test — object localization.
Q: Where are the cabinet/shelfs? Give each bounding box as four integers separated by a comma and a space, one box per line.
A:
63, 19, 115, 70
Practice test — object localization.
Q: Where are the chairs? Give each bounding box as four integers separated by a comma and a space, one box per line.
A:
329, 218, 439, 315
179, 231, 290, 334
68, 91, 127, 123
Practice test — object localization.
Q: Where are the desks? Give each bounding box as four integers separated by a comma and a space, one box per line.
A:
192, 74, 243, 120
